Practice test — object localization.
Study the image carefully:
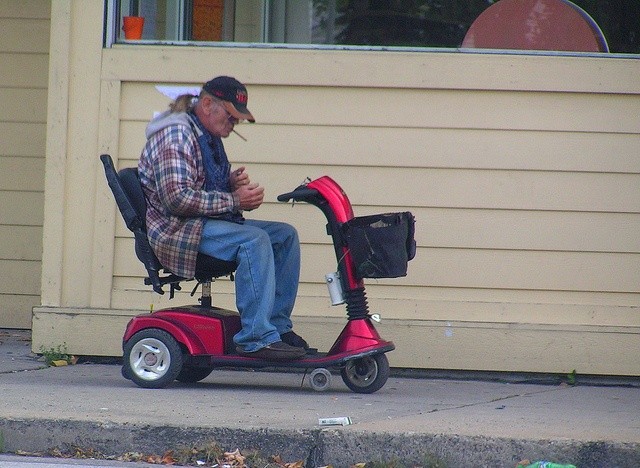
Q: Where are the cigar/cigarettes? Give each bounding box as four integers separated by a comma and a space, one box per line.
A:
232, 130, 247, 142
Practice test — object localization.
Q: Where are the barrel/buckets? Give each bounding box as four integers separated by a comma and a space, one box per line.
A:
123, 16, 144, 39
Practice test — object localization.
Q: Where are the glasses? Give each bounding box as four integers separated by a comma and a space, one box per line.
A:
211, 98, 239, 127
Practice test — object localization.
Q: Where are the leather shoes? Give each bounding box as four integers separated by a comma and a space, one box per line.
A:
237, 341, 306, 359
277, 331, 309, 351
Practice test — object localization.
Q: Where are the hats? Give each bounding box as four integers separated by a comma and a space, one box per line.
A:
201, 75, 255, 123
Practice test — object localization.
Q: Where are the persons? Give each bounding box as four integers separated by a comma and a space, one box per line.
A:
137, 76, 319, 362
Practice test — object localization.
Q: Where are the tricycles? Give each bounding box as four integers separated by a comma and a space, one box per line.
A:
101, 153, 417, 393
